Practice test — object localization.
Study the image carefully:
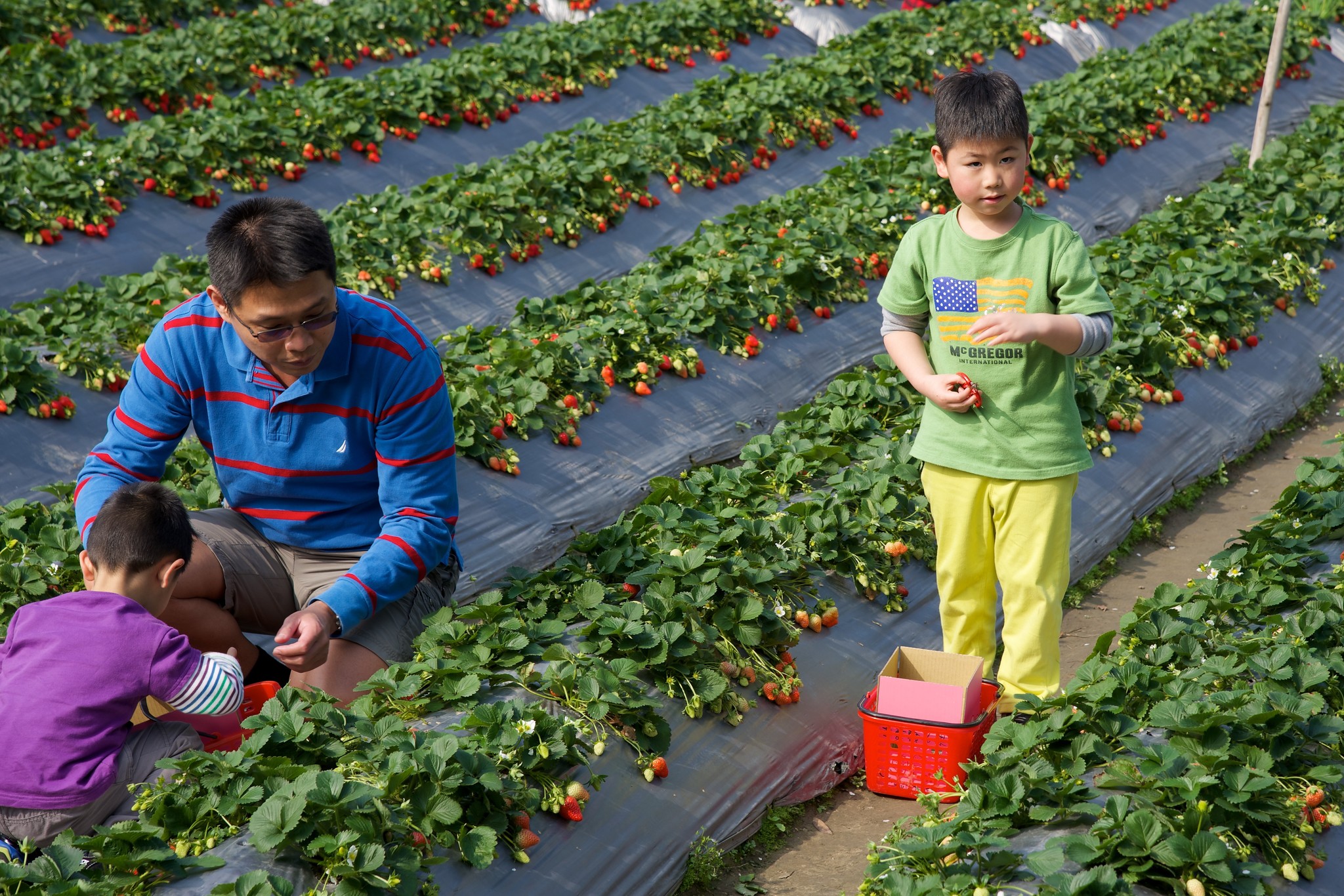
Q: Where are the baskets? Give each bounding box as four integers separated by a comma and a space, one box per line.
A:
131, 678, 283, 753
857, 667, 1005, 804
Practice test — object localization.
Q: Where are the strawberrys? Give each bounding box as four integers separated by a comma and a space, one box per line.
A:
0, 3, 1342, 896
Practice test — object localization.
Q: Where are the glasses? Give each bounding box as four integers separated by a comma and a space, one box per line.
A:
222, 280, 340, 344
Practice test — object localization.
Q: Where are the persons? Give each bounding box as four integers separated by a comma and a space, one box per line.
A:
874, 69, 1118, 729
69, 198, 463, 725
0, 477, 246, 859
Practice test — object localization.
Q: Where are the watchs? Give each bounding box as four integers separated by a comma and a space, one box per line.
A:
333, 611, 343, 631
